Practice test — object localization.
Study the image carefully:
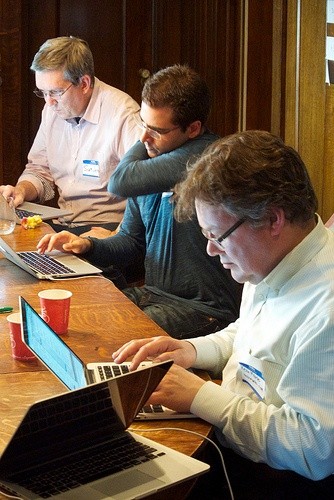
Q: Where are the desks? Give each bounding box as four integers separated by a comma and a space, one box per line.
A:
0, 217, 217, 500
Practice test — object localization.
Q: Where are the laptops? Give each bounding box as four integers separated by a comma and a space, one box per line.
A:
19, 295, 215, 420
0, 237, 104, 279
0, 358, 213, 500
0, 191, 74, 224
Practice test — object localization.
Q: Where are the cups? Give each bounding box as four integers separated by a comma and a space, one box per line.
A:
38, 289, 73, 335
6, 313, 43, 361
0, 196, 16, 236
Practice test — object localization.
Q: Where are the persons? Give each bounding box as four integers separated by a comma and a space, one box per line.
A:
1, 35, 145, 228
112, 130, 333, 500
35, 63, 243, 336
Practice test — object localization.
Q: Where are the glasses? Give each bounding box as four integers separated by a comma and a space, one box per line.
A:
134, 109, 183, 140
201, 216, 251, 251
33, 83, 73, 99
